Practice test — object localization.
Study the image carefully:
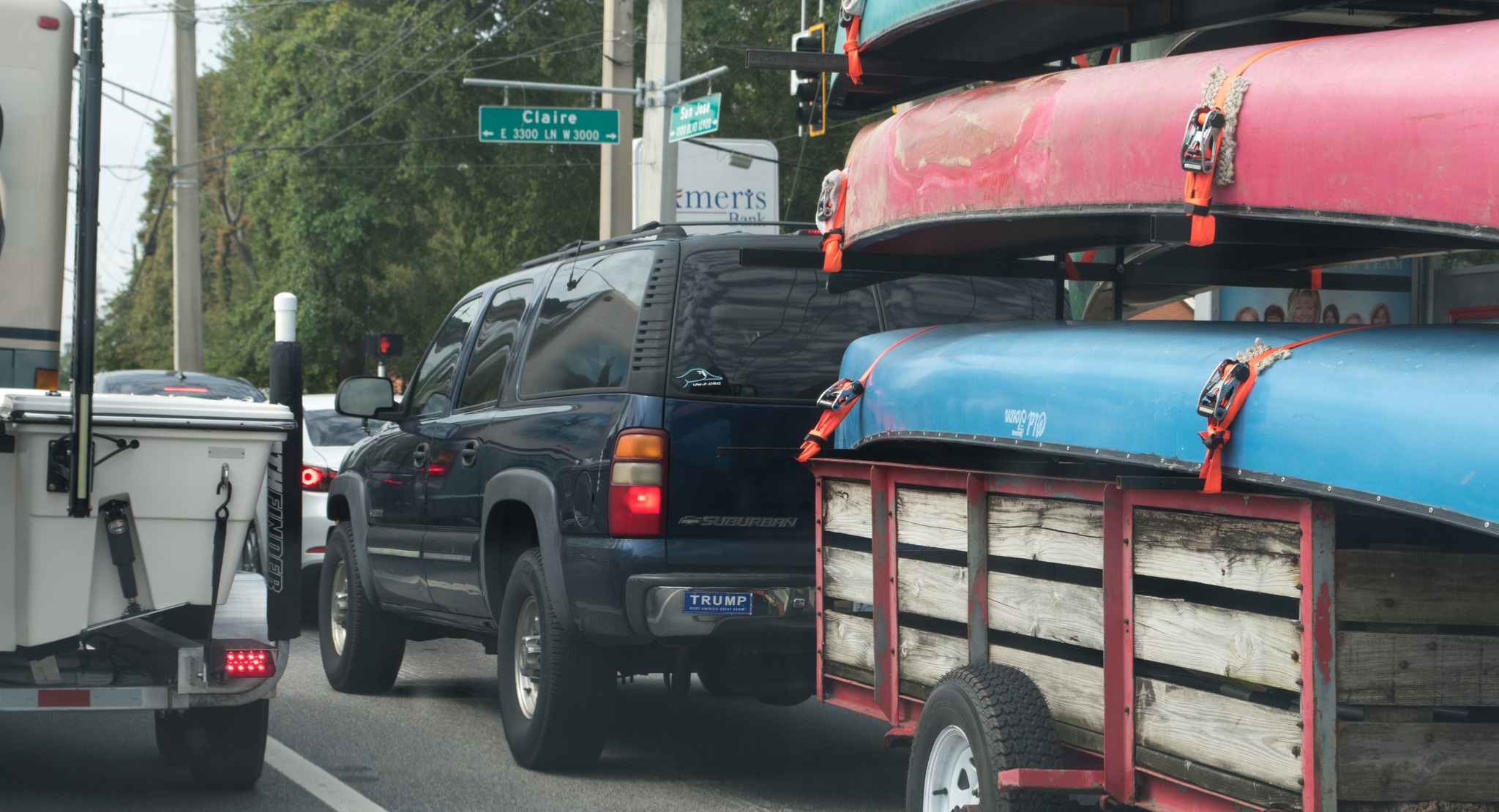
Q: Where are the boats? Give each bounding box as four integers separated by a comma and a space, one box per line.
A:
830, 320, 1499, 539
820, 2, 1499, 141
818, 17, 1499, 297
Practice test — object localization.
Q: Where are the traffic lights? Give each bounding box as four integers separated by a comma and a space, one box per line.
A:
790, 24, 830, 137
373, 332, 410, 363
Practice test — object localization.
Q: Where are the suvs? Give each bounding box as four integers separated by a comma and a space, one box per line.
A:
315, 229, 1070, 774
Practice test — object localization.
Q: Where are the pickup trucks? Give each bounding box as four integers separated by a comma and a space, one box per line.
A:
1, 387, 299, 800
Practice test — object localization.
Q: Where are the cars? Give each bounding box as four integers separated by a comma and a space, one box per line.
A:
94, 371, 268, 404
304, 395, 403, 591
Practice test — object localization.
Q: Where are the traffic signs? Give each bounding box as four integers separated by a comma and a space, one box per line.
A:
478, 105, 621, 146
669, 92, 721, 142
